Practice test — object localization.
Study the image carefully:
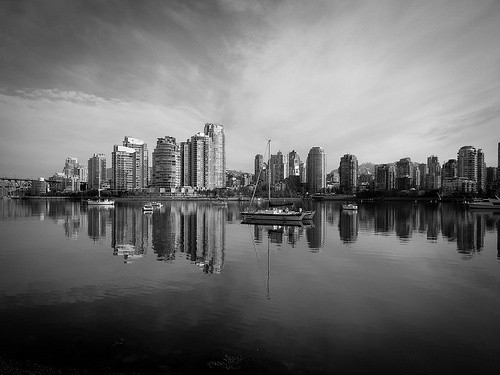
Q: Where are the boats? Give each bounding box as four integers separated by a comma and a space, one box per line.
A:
210, 199, 227, 205
151, 202, 164, 206
141, 205, 154, 211
425, 199, 442, 208
343, 203, 358, 210
466, 196, 500, 209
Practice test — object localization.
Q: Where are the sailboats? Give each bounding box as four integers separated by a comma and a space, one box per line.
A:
239, 139, 317, 221
240, 218, 315, 301
82, 172, 115, 205
